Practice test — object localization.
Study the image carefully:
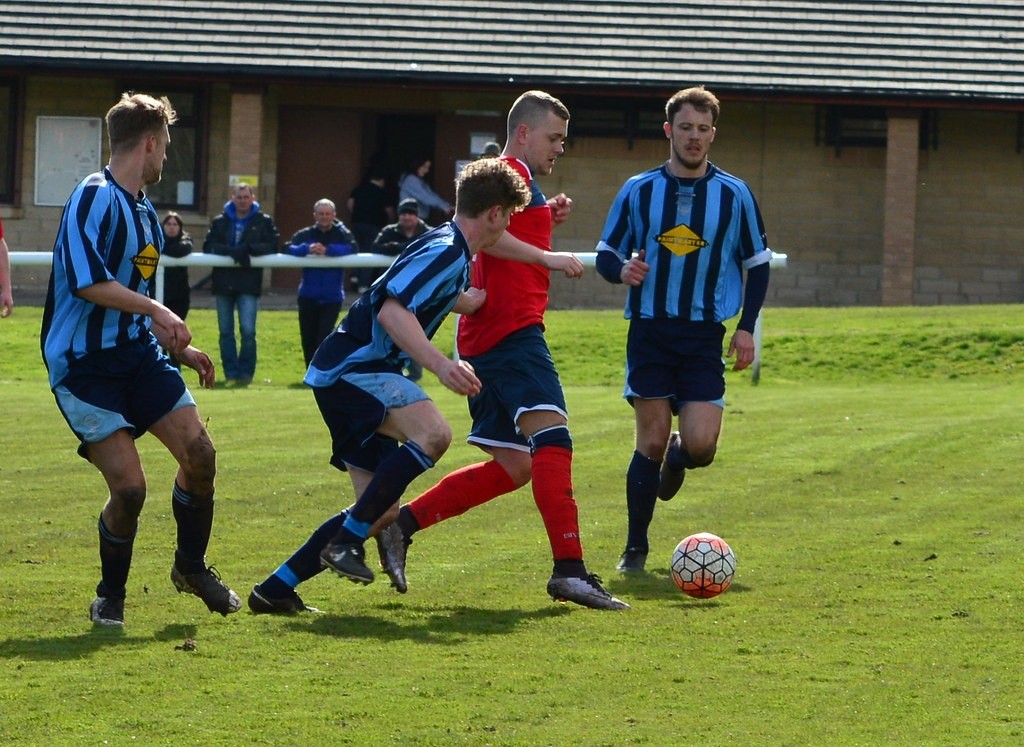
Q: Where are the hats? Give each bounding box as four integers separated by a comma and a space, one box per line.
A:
397, 199, 418, 215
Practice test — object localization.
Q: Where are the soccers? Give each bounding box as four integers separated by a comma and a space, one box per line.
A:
669, 532, 737, 599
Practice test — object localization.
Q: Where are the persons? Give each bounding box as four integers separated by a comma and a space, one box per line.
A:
479, 143, 501, 157
596, 87, 772, 571
373, 91, 629, 611
162, 212, 194, 371
0, 217, 14, 318
247, 158, 531, 617
202, 184, 280, 385
282, 198, 358, 369
373, 199, 433, 277
40, 95, 242, 624
347, 171, 396, 244
398, 154, 456, 218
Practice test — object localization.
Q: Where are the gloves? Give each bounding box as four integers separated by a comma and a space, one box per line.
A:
387, 241, 402, 256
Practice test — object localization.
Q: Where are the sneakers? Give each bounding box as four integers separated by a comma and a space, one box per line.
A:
658, 431, 685, 500
376, 521, 413, 593
91, 581, 127, 626
547, 573, 630, 610
617, 547, 646, 573
320, 536, 375, 587
170, 563, 242, 617
248, 584, 318, 614
